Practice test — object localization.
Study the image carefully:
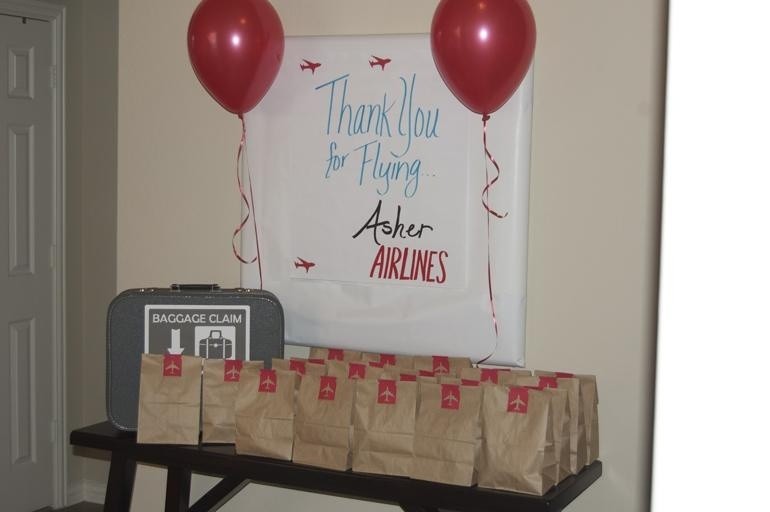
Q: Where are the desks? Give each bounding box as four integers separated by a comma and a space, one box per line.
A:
68, 417, 604, 511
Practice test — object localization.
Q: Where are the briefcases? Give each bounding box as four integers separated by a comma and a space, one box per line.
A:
105, 283, 284, 432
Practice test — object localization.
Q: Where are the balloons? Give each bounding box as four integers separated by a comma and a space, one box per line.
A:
187, 0, 285, 115
429, 0, 537, 115
